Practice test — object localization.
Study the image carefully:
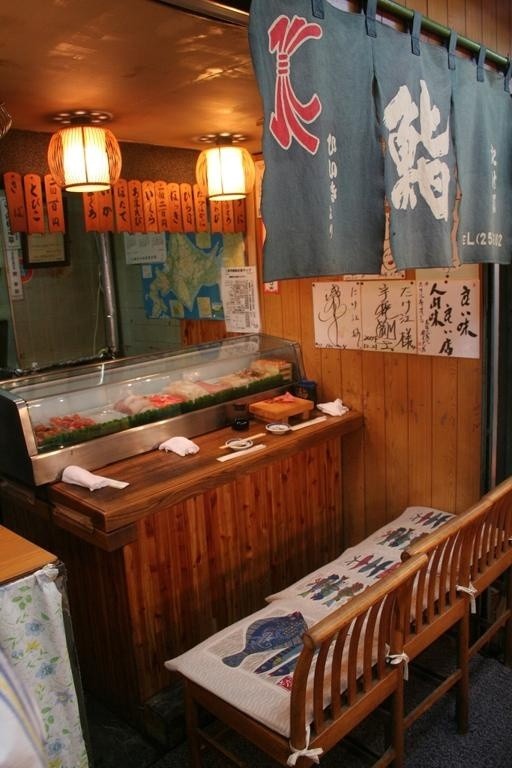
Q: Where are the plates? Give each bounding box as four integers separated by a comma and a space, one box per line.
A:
225, 438, 254, 451
265, 422, 292, 435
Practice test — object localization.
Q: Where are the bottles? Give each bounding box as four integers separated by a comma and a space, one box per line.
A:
233, 403, 249, 431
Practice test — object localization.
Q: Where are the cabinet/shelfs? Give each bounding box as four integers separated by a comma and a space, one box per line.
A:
47, 399, 365, 695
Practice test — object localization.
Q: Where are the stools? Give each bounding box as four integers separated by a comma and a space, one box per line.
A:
264, 498, 492, 734
165, 553, 427, 768
340, 477, 512, 659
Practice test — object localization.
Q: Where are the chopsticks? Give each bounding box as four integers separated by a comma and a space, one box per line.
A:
288, 415, 329, 433
211, 444, 267, 462
219, 433, 266, 450
